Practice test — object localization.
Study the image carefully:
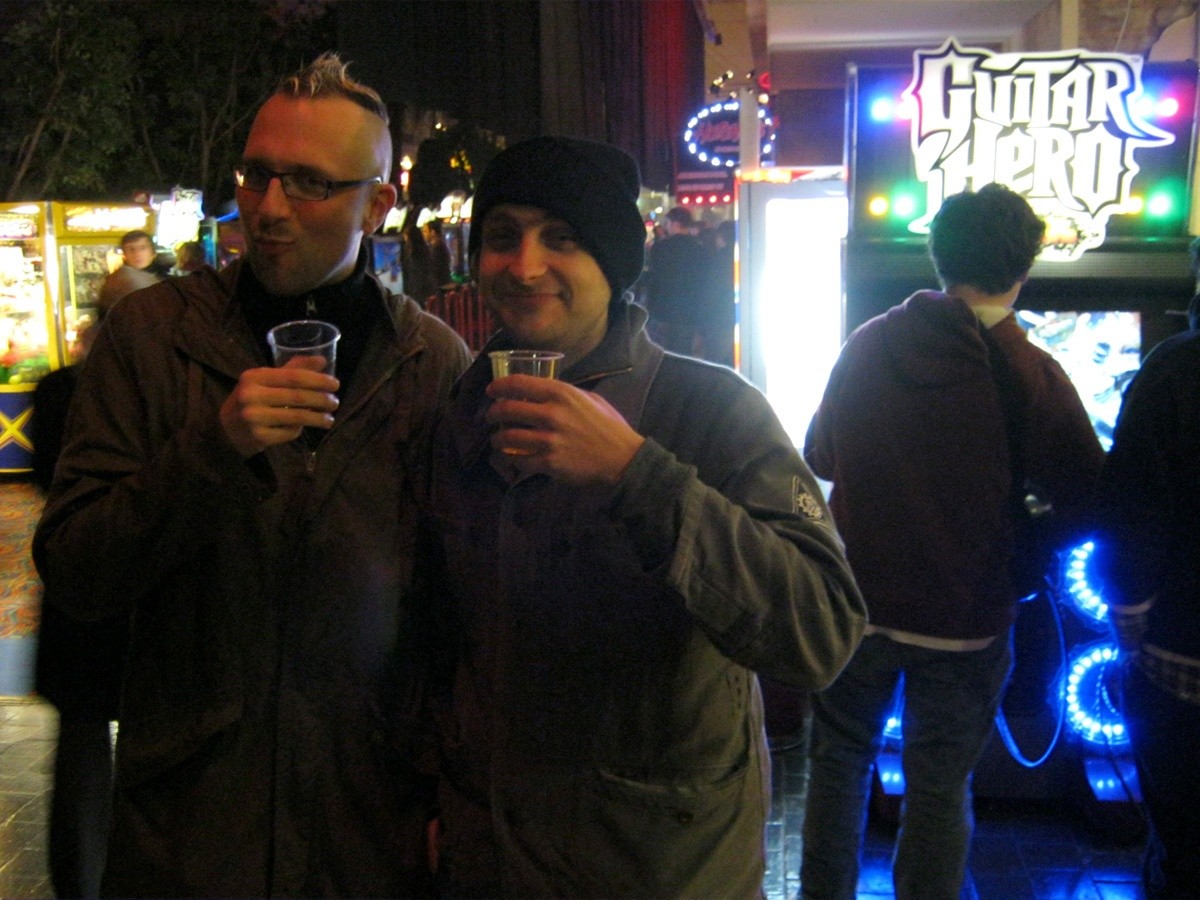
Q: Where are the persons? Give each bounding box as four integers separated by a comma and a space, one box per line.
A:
629, 206, 736, 372
32, 50, 471, 900
388, 134, 868, 900
422, 217, 453, 295
799, 182, 1107, 900
1107, 238, 1200, 900
121, 230, 175, 282
32, 265, 162, 900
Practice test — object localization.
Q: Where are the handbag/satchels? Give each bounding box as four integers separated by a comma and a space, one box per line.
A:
996, 497, 1056, 604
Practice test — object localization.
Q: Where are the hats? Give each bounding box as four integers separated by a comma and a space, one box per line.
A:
472, 136, 647, 301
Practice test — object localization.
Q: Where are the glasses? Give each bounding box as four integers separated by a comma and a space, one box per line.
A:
232, 164, 383, 202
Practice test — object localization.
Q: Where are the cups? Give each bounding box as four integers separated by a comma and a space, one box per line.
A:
266, 320, 342, 415
488, 349, 566, 455
